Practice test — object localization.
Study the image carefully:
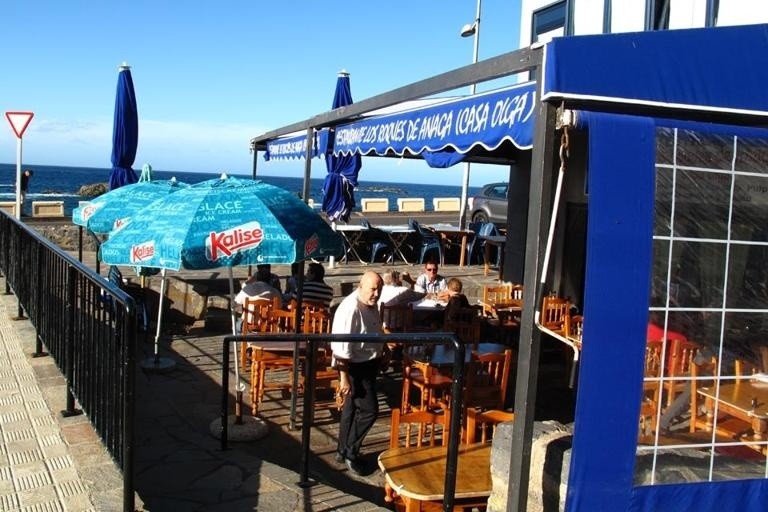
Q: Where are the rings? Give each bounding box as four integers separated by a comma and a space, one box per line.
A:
342, 388, 346, 393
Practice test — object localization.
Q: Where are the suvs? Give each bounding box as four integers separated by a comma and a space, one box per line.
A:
472, 181, 510, 229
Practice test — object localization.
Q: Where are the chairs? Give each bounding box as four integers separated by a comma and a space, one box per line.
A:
106, 265, 152, 345
330, 219, 508, 278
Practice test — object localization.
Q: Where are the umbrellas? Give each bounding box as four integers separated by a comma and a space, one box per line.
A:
133, 162, 160, 289
100, 173, 344, 390
73, 176, 192, 356
110, 61, 140, 189
322, 70, 361, 266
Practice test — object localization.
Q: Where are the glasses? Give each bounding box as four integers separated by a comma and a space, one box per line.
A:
426, 268, 437, 272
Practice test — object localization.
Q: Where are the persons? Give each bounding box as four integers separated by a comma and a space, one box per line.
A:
15, 169, 34, 217
233, 260, 499, 360
329, 270, 403, 476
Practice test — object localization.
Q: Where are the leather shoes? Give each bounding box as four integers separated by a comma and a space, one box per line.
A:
335, 453, 346, 463
345, 456, 365, 476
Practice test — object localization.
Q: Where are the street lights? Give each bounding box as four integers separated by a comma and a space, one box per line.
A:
452, 2, 483, 230
